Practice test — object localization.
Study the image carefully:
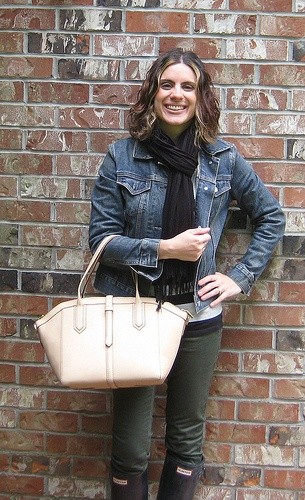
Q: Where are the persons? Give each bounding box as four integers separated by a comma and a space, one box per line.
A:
87, 47, 286, 500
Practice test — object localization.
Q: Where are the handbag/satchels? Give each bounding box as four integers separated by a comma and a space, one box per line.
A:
33, 235, 193, 390
